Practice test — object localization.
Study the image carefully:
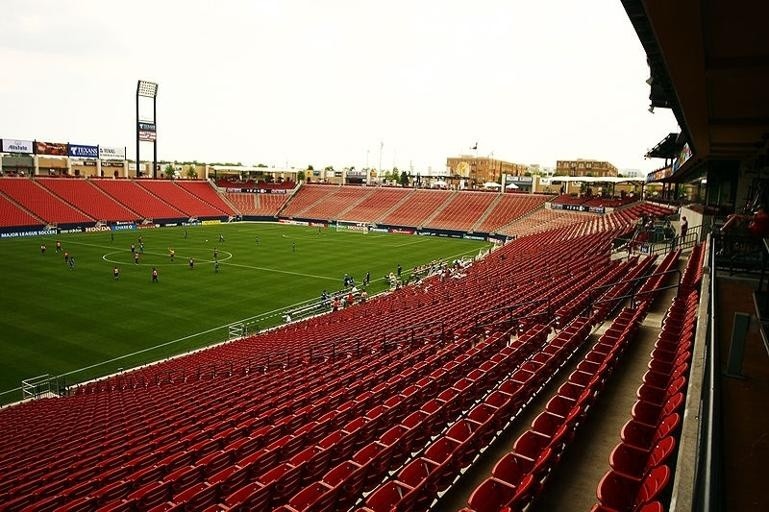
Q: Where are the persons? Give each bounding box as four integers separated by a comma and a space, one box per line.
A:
41, 226, 295, 283
321, 257, 471, 312
560, 185, 769, 258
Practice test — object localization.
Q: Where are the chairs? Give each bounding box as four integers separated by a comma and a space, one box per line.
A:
0, 203, 707, 512
